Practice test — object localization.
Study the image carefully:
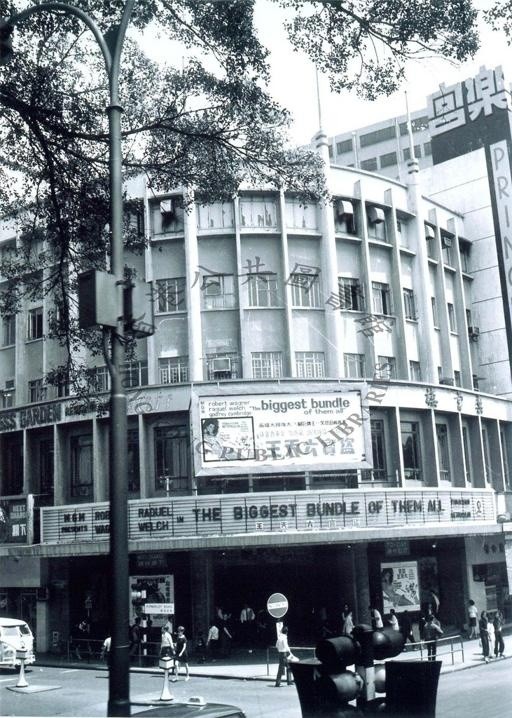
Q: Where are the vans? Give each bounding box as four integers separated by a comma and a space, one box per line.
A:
0, 616, 36, 670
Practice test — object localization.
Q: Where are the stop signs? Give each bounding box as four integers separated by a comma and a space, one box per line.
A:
266, 591, 288, 617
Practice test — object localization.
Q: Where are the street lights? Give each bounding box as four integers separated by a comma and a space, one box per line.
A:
1, 0, 134, 714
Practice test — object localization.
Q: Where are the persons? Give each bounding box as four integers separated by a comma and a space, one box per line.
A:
159, 614, 190, 682
72, 615, 93, 661
466, 599, 507, 667
196, 604, 257, 663
100, 634, 113, 677
382, 567, 414, 607
319, 603, 333, 635
339, 604, 357, 638
203, 419, 253, 461
272, 627, 298, 688
129, 617, 141, 665
367, 590, 444, 665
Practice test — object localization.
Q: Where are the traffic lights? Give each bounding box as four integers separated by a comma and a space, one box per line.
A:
315, 635, 362, 717
362, 629, 405, 717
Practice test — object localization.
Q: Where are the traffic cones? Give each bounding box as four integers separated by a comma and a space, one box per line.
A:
14, 658, 28, 686
